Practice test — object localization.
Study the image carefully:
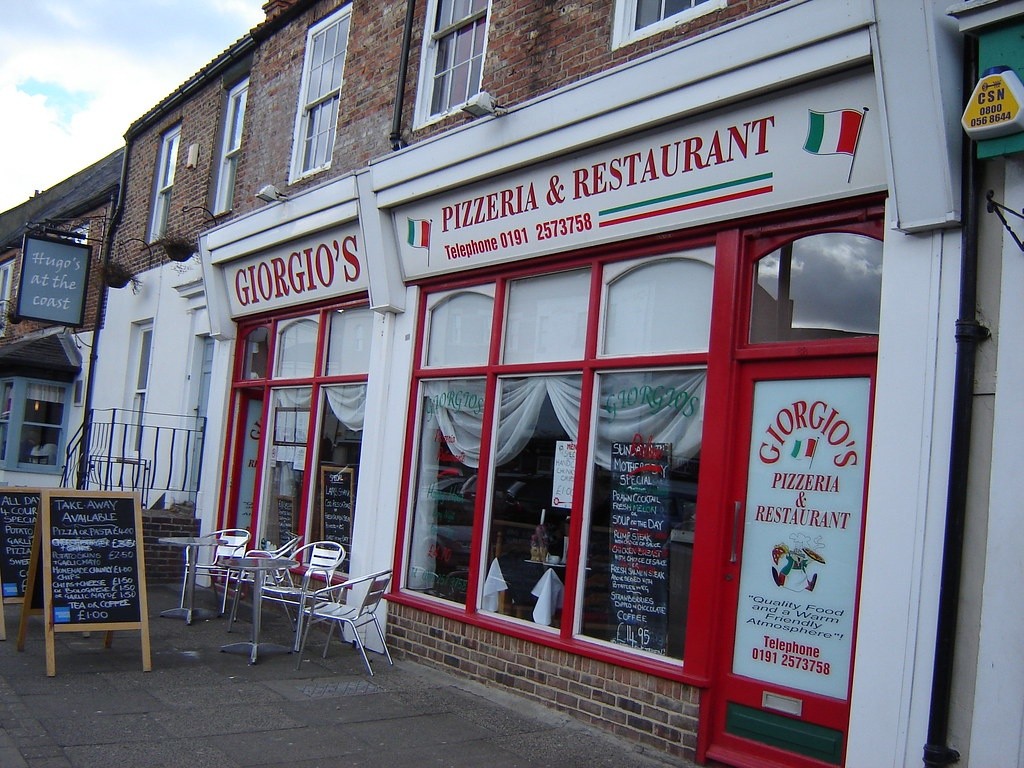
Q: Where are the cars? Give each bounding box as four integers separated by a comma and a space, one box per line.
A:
460, 473, 528, 500
427, 494, 478, 575
422, 477, 466, 495
427, 487, 465, 525
644, 469, 698, 518
505, 471, 561, 501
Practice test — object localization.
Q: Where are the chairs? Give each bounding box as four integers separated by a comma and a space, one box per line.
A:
180, 528, 252, 614
227, 535, 305, 634
259, 541, 345, 651
295, 568, 393, 677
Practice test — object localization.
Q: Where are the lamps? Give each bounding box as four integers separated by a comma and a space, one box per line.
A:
459, 91, 507, 119
255, 184, 288, 204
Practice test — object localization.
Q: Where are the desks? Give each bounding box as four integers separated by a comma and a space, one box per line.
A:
159, 537, 229, 625
523, 558, 592, 629
216, 558, 300, 666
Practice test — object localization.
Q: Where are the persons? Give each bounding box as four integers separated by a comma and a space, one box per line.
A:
556, 518, 570, 555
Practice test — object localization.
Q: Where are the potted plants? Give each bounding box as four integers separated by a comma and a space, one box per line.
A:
89, 258, 143, 296
0, 298, 31, 331
154, 225, 200, 277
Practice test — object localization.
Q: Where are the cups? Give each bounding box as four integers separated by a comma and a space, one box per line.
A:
549, 556, 560, 564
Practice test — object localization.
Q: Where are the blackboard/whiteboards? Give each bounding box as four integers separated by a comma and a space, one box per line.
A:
275, 495, 296, 558
0, 488, 43, 604
321, 466, 355, 563
611, 442, 672, 656
42, 488, 148, 632
